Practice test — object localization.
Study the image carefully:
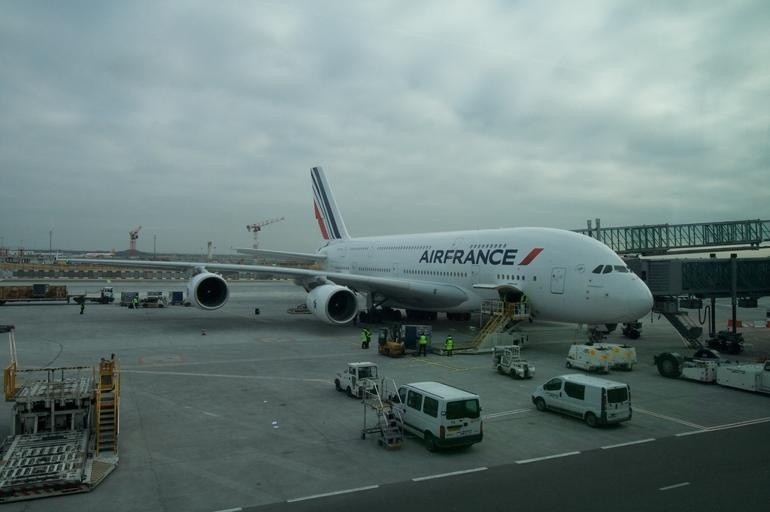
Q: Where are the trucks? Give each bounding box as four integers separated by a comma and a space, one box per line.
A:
654, 349, 770, 395
1, 283, 116, 306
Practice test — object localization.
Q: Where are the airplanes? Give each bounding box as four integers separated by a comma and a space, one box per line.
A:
54, 163, 770, 357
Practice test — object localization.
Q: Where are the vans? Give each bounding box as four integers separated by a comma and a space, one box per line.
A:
529, 373, 633, 428
565, 343, 614, 373
593, 342, 638, 372
393, 379, 485, 453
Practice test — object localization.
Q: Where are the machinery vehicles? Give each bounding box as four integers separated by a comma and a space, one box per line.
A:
378, 322, 407, 358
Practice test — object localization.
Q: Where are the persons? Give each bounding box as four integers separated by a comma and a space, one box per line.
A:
80, 302, 85, 314
445, 334, 454, 356
584, 335, 594, 346
377, 326, 388, 346
416, 332, 428, 357
360, 326, 371, 349
129, 292, 139, 309
